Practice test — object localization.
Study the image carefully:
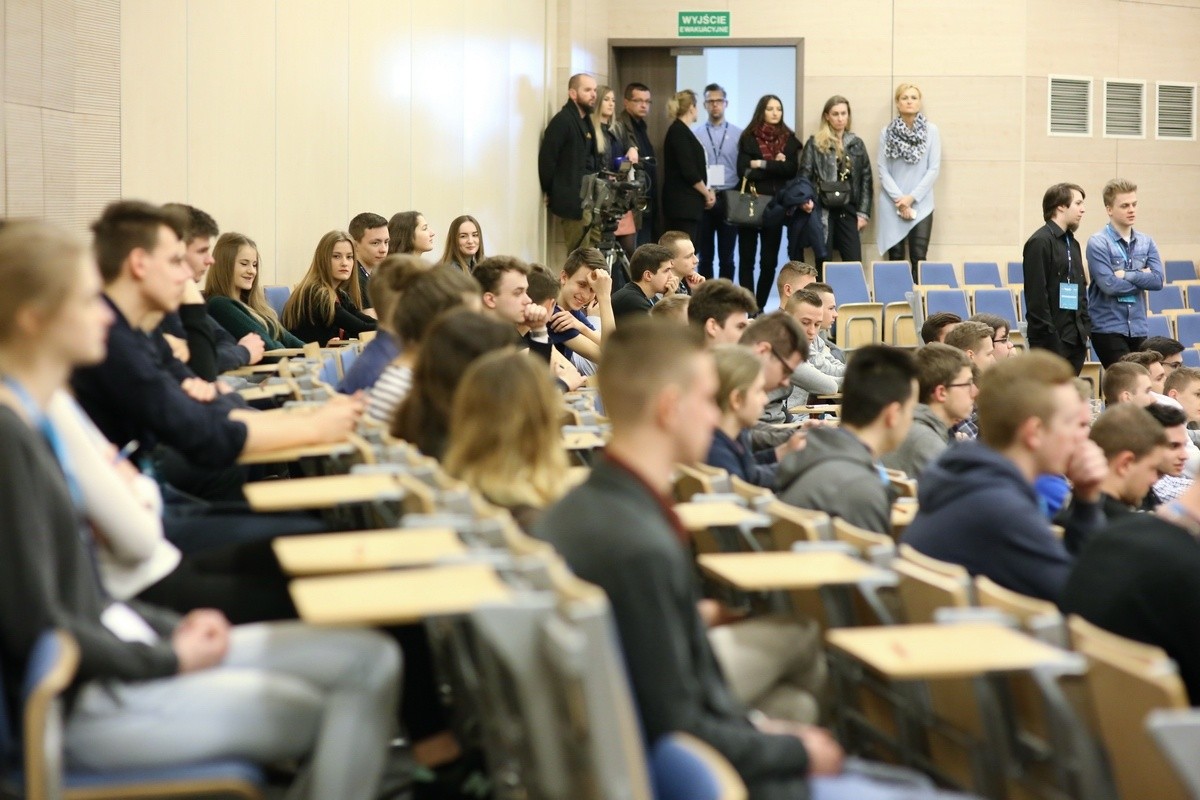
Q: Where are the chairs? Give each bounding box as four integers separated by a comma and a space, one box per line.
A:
19, 260, 1200, 800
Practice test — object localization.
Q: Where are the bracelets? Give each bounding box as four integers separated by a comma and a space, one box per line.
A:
530, 330, 547, 337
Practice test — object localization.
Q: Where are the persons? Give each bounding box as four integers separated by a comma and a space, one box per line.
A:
691, 83, 744, 283
662, 90, 716, 241
799, 95, 873, 261
0, 199, 1200, 800
1022, 182, 1090, 378
737, 95, 803, 318
876, 82, 940, 284
541, 73, 601, 256
617, 83, 660, 246
589, 84, 636, 257
1086, 178, 1164, 367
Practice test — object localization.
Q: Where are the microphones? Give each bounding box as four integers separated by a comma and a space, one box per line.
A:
612, 182, 635, 190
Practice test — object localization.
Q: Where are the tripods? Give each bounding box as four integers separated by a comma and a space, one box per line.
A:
571, 216, 633, 285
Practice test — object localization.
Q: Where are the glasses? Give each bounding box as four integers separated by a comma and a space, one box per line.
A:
630, 99, 651, 105
993, 334, 1010, 342
705, 99, 724, 106
944, 377, 974, 388
758, 342, 795, 380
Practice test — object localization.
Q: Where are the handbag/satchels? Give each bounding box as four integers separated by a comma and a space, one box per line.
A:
820, 179, 851, 206
724, 176, 773, 228
